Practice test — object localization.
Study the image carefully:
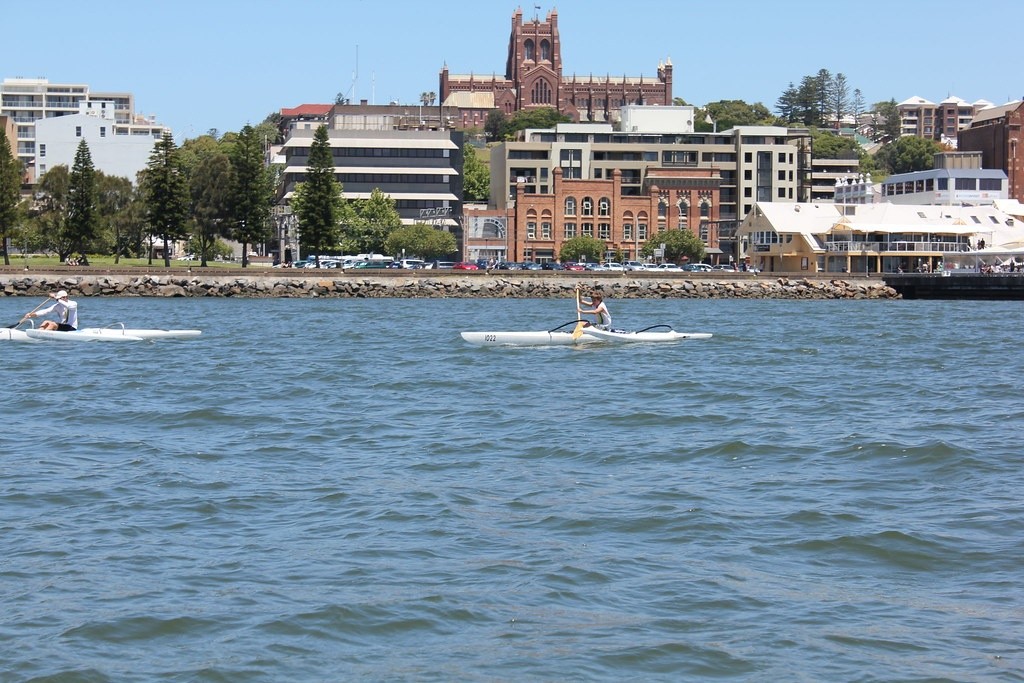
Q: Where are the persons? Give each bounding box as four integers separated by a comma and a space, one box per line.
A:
759, 261, 764, 272
73, 256, 79, 266
574, 283, 611, 331
64, 254, 74, 265
274, 258, 292, 268
732, 260, 737, 272
891, 235, 1024, 276
78, 251, 89, 266
25, 291, 78, 331
743, 260, 746, 272
488, 256, 493, 265
491, 257, 499, 269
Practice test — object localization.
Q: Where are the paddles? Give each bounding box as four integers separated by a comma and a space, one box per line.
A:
573, 287, 583, 339
6, 298, 50, 329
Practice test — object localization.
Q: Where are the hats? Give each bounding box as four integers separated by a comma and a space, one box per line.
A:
590, 292, 602, 298
56, 290, 67, 299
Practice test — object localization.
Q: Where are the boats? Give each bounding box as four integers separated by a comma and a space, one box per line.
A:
582, 324, 714, 342
1, 320, 202, 341
461, 320, 590, 346
26, 328, 143, 343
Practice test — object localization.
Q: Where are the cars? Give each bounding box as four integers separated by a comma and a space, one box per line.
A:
272, 254, 761, 274
